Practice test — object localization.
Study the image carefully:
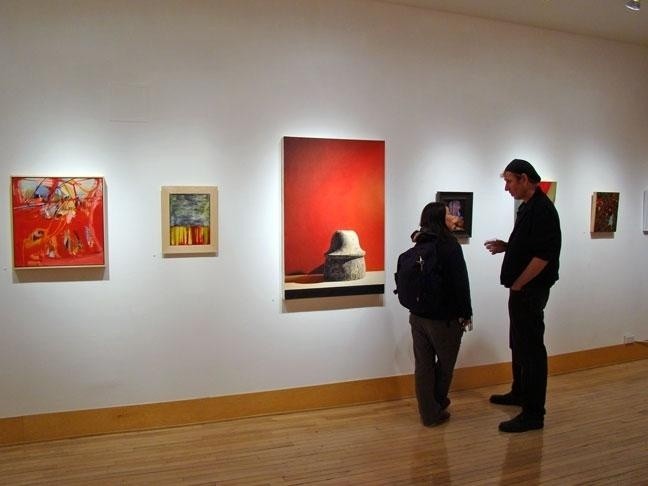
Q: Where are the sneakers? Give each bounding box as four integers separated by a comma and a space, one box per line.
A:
422, 397, 452, 427
489, 390, 519, 405
498, 411, 544, 432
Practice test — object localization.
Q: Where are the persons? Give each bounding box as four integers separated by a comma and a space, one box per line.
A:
408, 201, 474, 427
483, 158, 563, 434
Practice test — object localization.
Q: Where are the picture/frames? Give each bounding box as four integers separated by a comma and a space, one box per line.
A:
9, 175, 107, 271
161, 185, 219, 255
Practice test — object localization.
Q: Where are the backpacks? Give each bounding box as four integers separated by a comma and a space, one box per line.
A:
394, 236, 431, 310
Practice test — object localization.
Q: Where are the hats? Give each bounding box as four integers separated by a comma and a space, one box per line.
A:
505, 159, 541, 181
